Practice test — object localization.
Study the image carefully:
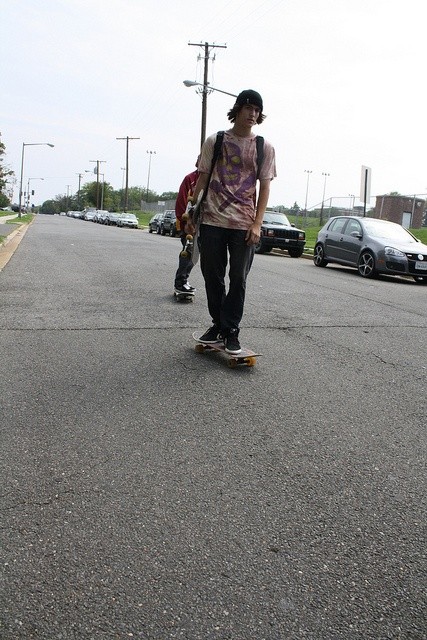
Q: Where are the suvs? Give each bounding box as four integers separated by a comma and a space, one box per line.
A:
253, 211, 307, 258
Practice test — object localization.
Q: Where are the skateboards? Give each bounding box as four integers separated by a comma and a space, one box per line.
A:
192, 328, 263, 368
173, 289, 195, 303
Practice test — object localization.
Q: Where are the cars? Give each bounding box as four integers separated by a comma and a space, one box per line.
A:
104, 212, 121, 226
314, 214, 427, 285
160, 210, 178, 236
148, 214, 163, 234
58, 206, 108, 224
117, 213, 140, 228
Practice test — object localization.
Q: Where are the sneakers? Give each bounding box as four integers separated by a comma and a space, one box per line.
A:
186, 282, 196, 291
221, 328, 241, 354
197, 320, 223, 342
174, 283, 194, 294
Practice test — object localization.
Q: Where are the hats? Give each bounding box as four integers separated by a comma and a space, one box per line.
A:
235, 90, 263, 112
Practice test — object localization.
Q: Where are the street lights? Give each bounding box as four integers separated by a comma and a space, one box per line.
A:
146, 151, 157, 203
17, 142, 55, 219
304, 170, 313, 228
26, 177, 44, 214
84, 170, 104, 209
320, 172, 331, 225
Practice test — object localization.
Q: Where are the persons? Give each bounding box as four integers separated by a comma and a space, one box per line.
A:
174, 154, 199, 293
186, 89, 277, 353
